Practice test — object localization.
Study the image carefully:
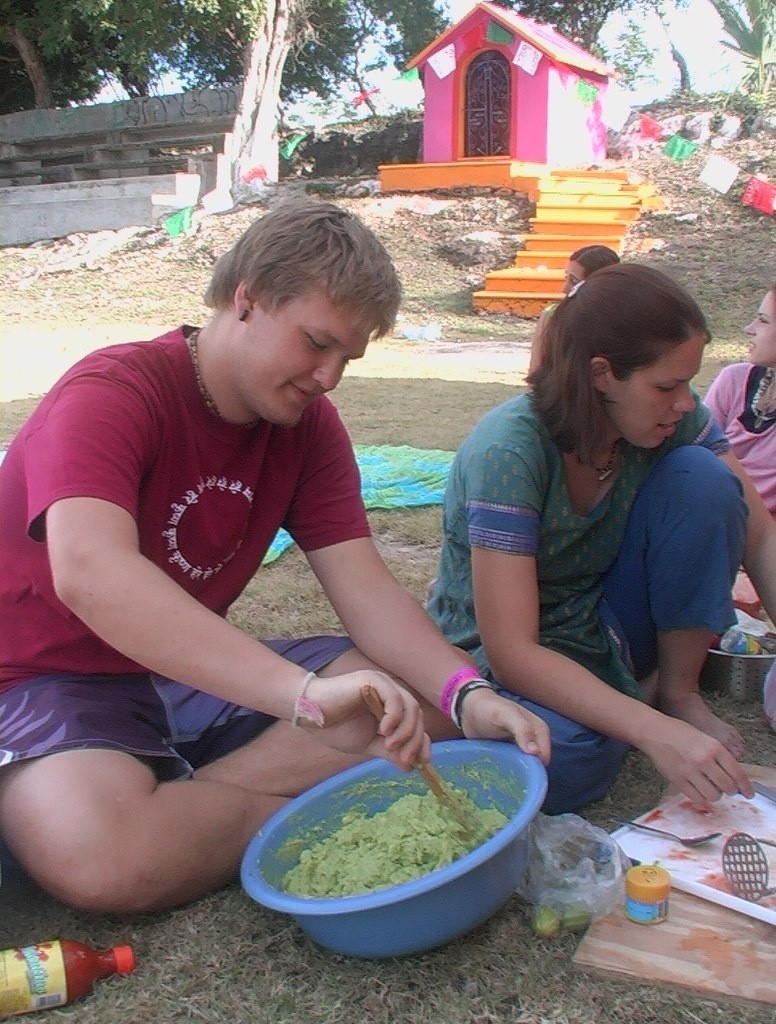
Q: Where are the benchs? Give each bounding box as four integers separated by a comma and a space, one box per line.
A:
0, 83, 244, 244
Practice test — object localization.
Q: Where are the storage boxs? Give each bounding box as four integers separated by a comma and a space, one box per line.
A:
699, 648, 776, 703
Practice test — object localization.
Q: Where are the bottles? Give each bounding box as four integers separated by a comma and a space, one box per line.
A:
0, 940, 136, 1015
720, 630, 768, 656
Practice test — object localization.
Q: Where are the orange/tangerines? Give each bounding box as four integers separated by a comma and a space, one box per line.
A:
530, 902, 587, 937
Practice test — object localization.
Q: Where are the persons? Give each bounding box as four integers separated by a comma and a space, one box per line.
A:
0, 207, 552, 910
428, 247, 776, 815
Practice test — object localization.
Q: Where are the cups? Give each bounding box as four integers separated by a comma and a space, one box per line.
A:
626, 863, 670, 923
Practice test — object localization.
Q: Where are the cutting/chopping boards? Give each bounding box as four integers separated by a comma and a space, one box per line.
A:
570, 763, 776, 1005
608, 787, 776, 925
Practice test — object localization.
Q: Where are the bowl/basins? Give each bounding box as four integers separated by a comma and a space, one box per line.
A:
239, 739, 546, 958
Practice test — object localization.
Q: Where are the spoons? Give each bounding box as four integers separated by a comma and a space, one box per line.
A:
607, 814, 720, 848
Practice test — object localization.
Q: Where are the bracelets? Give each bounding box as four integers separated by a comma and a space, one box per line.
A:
443, 668, 492, 727
294, 673, 316, 732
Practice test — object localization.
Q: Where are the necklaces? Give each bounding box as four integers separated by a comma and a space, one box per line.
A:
189, 335, 257, 427
761, 376, 776, 413
577, 440, 615, 480
